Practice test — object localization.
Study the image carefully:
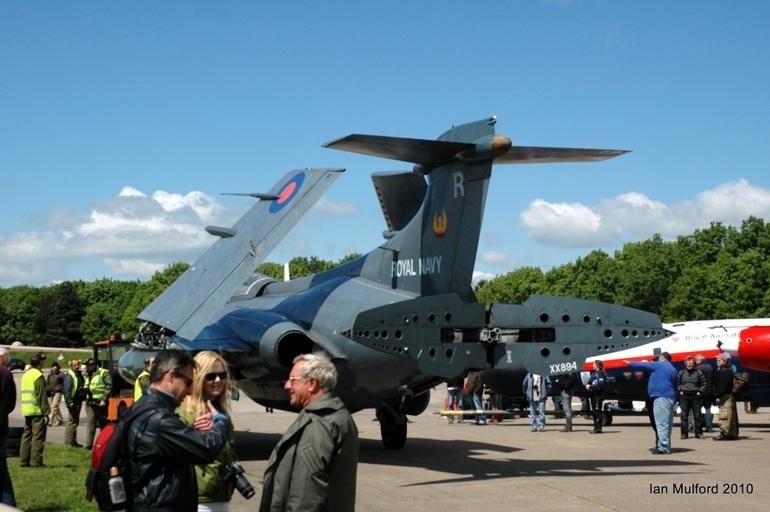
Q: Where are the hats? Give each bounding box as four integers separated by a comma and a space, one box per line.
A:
30, 351, 47, 361
715, 351, 732, 363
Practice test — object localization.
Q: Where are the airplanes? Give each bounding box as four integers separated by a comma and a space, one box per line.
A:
105, 102, 636, 463
434, 290, 770, 429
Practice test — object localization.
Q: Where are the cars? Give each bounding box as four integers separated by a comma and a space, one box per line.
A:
5, 358, 26, 372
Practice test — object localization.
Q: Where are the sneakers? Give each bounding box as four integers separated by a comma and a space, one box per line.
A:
66, 437, 94, 449
713, 435, 737, 441
558, 427, 603, 434
530, 428, 545, 433
648, 447, 665, 455
679, 433, 706, 441
447, 417, 499, 425
46, 418, 65, 427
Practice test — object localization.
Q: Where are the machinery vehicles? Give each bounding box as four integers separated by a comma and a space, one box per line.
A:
82, 332, 136, 423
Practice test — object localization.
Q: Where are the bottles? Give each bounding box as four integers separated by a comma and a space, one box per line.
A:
107, 465, 128, 512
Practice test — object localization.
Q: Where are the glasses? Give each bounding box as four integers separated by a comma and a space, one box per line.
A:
204, 371, 228, 382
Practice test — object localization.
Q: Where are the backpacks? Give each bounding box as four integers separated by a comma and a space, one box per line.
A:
85, 395, 166, 511
721, 369, 749, 393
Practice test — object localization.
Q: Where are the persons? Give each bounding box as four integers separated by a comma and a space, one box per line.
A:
623, 350, 679, 455
464, 366, 487, 425
174, 348, 242, 512
583, 359, 609, 434
555, 373, 575, 433
1, 344, 158, 512
675, 355, 705, 440
520, 368, 553, 432
710, 352, 735, 441
257, 349, 358, 510
121, 348, 234, 512
715, 350, 741, 439
479, 382, 493, 420
688, 352, 714, 433
444, 372, 466, 425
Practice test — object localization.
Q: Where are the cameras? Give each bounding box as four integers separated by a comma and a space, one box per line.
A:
220, 461, 255, 500
